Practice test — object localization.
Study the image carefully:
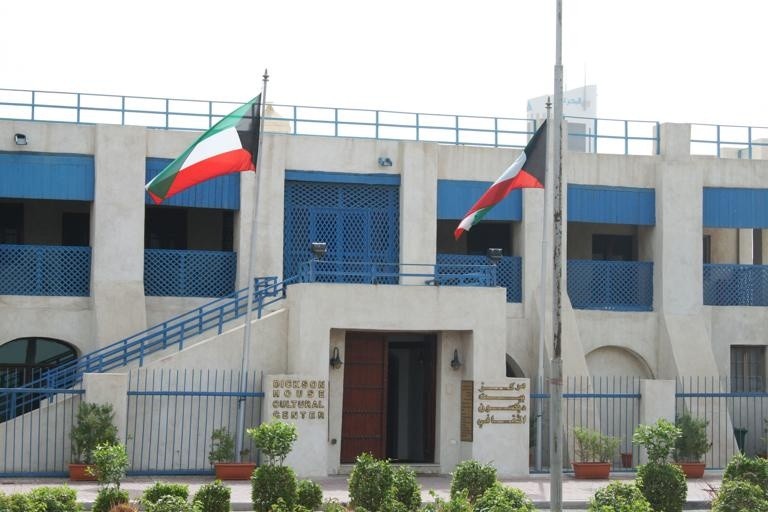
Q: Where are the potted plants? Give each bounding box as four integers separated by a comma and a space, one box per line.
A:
566, 422, 622, 479
62, 396, 123, 485
207, 424, 257, 482
666, 409, 714, 479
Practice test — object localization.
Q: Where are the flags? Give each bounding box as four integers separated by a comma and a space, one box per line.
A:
454, 118, 547, 241
144, 92, 262, 208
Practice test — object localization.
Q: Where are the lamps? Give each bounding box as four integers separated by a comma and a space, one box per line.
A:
450, 347, 463, 372
329, 345, 343, 372
